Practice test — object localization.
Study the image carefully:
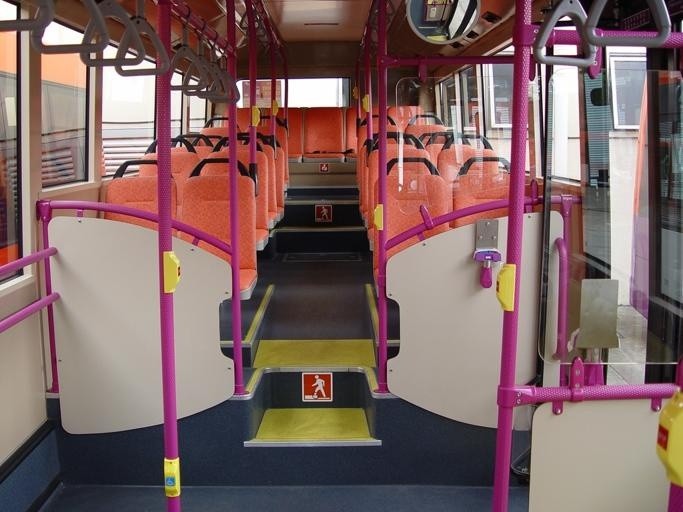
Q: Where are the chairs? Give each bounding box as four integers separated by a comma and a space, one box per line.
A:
103, 102, 302, 303
302, 106, 344, 164
345, 102, 512, 301
